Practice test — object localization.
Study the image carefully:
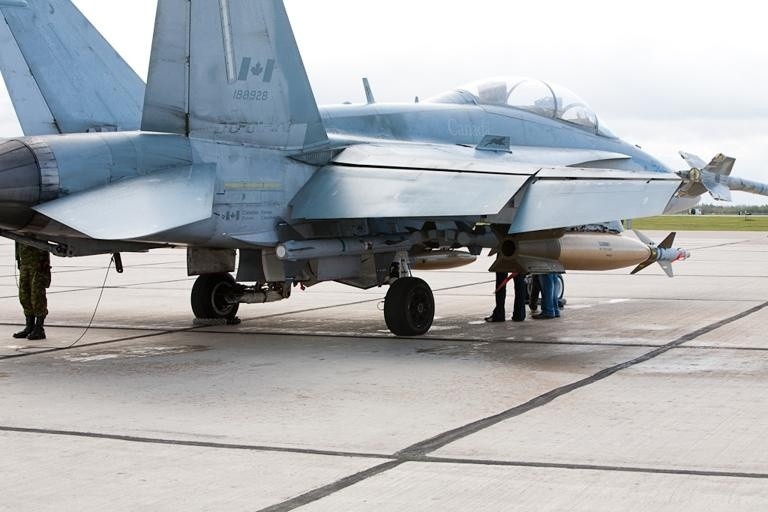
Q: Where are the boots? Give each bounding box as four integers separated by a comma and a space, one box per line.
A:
13, 315, 35, 338
26, 317, 46, 340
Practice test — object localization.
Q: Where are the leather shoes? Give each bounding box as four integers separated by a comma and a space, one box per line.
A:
531, 311, 544, 319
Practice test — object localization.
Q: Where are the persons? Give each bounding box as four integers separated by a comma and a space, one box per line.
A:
529, 274, 544, 313
484, 273, 526, 322
13, 243, 52, 340
532, 273, 560, 319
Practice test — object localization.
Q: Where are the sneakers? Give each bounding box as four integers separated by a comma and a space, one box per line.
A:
484, 316, 494, 322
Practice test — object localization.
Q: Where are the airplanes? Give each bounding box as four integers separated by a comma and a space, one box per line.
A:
0, 0, 767, 339
